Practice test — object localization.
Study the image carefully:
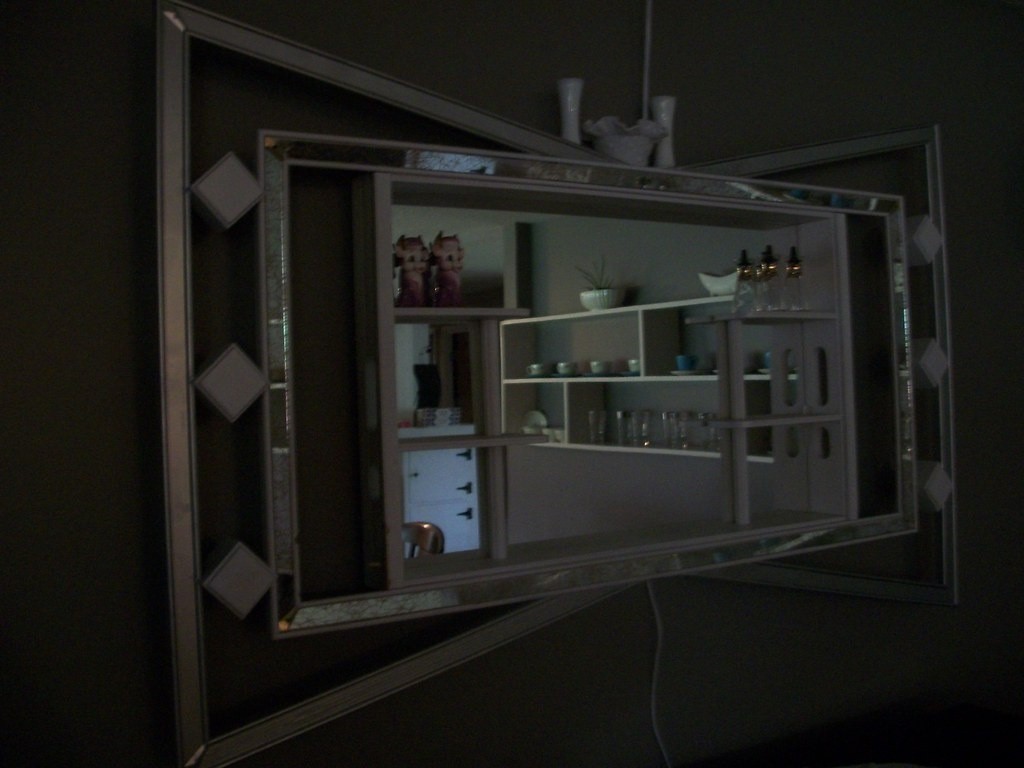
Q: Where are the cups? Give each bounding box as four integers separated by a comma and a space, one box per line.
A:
616, 411, 722, 451
627, 359, 641, 372
589, 361, 613, 373
526, 364, 544, 374
588, 410, 608, 445
555, 362, 578, 374
674, 355, 691, 371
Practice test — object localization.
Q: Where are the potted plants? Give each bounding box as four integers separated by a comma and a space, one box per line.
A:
572, 251, 626, 312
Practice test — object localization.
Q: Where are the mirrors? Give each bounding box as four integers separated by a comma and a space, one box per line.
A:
256, 129, 921, 644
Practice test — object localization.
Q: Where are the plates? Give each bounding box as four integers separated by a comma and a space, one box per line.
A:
618, 371, 640, 376
757, 368, 772, 374
583, 373, 615, 377
670, 370, 699, 375
551, 374, 581, 377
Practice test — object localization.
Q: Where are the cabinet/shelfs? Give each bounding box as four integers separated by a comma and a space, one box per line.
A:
499, 292, 807, 466
390, 215, 550, 560
682, 308, 842, 524
